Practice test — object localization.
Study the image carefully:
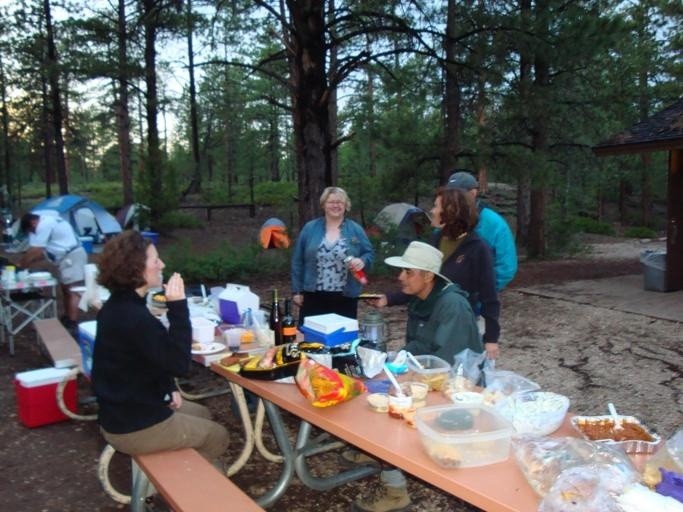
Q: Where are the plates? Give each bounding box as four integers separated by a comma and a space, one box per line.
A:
190, 342, 225, 355
32, 272, 52, 281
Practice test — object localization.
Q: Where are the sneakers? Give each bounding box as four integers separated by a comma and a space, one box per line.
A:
352, 486, 411, 511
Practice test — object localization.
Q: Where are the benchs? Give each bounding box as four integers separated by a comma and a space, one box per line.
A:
33, 317, 83, 368
81, 373, 265, 511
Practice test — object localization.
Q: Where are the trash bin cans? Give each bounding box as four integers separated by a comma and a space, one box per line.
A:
640, 251, 668, 292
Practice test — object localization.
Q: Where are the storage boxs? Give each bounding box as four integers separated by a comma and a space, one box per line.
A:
140, 231, 160, 248
79, 236, 94, 254
78, 320, 98, 378
299, 312, 359, 347
10, 367, 78, 429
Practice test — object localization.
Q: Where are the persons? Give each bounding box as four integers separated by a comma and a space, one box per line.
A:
16, 213, 89, 333
341, 240, 486, 510
92, 228, 231, 466
449, 172, 519, 334
289, 186, 374, 328
365, 186, 499, 361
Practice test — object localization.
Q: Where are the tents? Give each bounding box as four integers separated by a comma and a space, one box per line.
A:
116, 201, 152, 233
372, 202, 441, 249
12, 193, 122, 244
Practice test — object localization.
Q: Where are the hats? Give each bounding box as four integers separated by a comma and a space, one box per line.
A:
385, 241, 453, 284
444, 171, 480, 191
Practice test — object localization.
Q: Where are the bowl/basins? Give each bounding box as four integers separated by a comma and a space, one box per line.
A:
492, 391, 570, 438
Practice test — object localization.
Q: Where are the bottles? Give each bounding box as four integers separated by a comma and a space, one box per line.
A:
269, 290, 282, 346
359, 311, 391, 363
83, 264, 98, 290
282, 300, 297, 343
344, 255, 367, 285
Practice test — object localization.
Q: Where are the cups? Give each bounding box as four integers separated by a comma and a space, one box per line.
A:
411, 381, 429, 409
366, 392, 390, 413
226, 329, 241, 352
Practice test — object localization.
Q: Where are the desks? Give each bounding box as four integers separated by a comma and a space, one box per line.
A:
190, 329, 309, 367
209, 331, 682, 511
0, 277, 59, 357
